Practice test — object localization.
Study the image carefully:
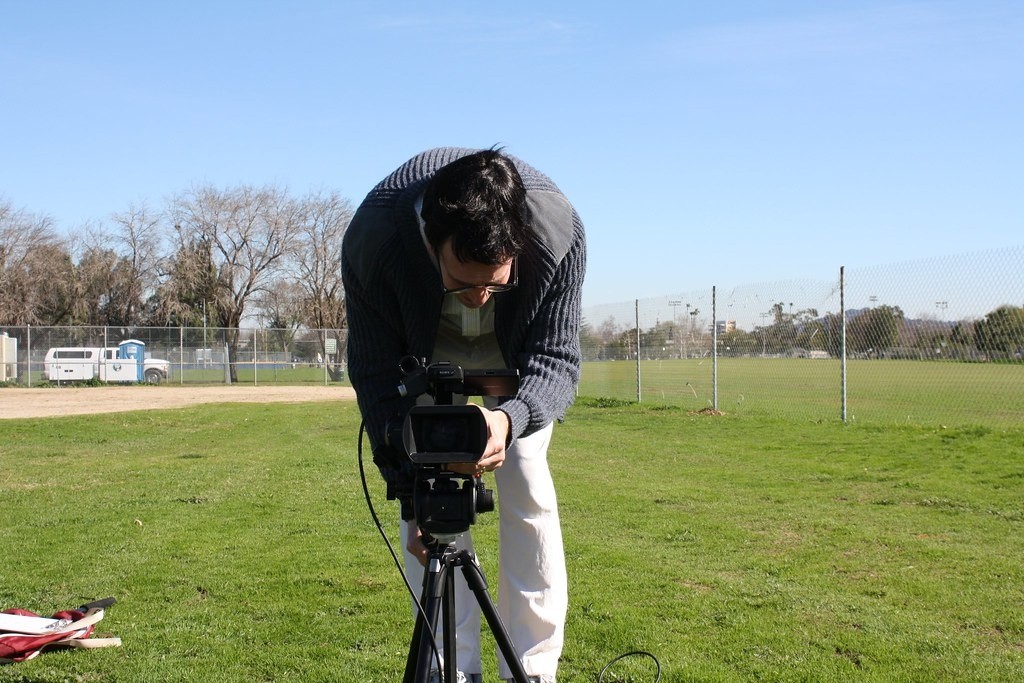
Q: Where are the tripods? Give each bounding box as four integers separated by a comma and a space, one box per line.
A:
402, 468, 532, 683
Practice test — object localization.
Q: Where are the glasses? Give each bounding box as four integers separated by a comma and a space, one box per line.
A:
433, 249, 518, 294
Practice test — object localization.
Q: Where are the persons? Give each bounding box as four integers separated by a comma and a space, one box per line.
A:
341, 146, 587, 683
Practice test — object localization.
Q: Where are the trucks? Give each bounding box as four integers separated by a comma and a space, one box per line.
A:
43, 346, 171, 386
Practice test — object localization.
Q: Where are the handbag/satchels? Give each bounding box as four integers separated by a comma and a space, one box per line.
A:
0, 607, 122, 661
